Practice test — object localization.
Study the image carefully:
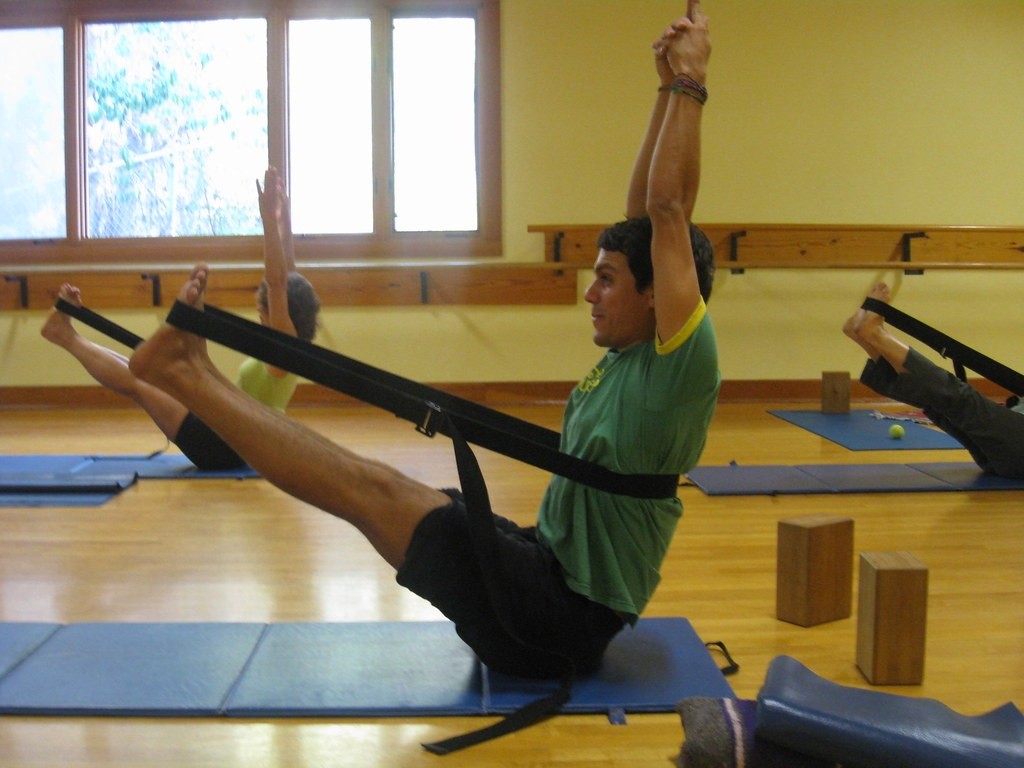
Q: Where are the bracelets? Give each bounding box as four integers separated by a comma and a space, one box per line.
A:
658, 84, 672, 92
670, 72, 708, 105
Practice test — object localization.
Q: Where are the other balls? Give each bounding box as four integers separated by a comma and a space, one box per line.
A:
889, 424, 905, 439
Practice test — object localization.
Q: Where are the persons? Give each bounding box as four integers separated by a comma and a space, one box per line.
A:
843, 282, 1024, 478
130, 0, 716, 678
41, 165, 321, 472
